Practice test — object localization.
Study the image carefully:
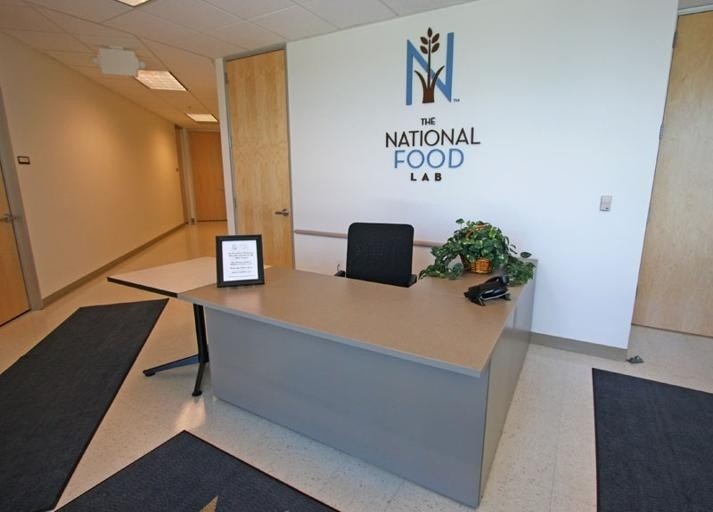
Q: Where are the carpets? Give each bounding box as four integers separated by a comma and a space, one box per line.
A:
590, 368, 713, 512
53, 431, 337, 512
0, 297, 172, 510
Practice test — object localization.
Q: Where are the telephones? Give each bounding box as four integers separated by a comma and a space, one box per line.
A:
464, 277, 511, 306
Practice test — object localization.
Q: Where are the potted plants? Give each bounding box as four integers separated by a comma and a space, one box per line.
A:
420, 220, 533, 282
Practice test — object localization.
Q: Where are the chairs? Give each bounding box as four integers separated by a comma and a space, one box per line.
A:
338, 224, 415, 290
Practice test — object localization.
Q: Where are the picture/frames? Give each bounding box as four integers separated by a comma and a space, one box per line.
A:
214, 234, 264, 288
